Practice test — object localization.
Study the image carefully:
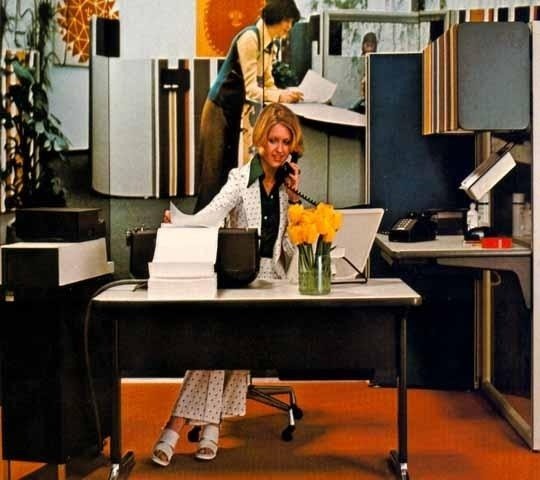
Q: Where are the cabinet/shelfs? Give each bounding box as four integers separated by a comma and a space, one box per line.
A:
0, 237, 119, 480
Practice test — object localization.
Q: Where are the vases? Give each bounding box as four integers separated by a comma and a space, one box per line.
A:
296, 238, 334, 295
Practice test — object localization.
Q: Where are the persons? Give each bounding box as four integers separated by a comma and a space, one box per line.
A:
357, 32, 377, 54
192, 0, 304, 223
151, 103, 303, 467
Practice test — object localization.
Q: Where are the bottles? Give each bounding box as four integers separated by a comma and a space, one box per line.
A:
513, 192, 526, 236
466, 203, 478, 231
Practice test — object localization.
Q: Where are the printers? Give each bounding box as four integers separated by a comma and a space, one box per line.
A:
129, 227, 260, 289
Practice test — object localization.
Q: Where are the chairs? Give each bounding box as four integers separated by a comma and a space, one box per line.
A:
185, 180, 304, 444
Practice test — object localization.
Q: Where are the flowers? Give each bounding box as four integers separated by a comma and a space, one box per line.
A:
284, 197, 348, 284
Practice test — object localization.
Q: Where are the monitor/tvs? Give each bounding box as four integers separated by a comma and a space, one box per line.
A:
287, 208, 385, 284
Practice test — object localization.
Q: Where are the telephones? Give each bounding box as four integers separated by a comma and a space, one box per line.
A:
274, 152, 298, 180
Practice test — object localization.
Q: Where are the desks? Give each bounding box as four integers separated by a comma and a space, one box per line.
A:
93, 271, 426, 475
370, 230, 535, 453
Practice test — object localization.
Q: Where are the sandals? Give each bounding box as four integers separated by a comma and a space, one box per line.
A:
152, 429, 180, 466
195, 424, 219, 460
